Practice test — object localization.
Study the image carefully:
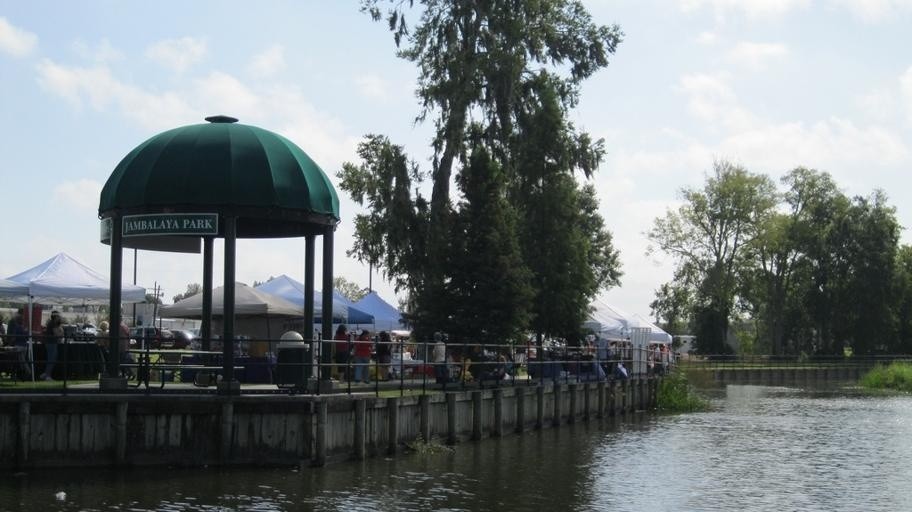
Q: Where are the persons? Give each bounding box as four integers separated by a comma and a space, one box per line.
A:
335, 326, 392, 382
39, 311, 63, 381
431, 331, 667, 383
97, 321, 109, 348
120, 317, 130, 377
13, 318, 31, 376
7, 309, 24, 346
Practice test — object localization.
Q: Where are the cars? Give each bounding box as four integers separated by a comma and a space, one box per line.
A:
128, 326, 200, 349
